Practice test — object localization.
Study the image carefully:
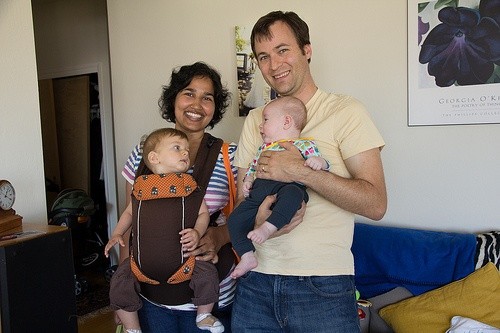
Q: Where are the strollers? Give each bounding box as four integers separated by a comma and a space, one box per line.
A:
48, 187, 119, 299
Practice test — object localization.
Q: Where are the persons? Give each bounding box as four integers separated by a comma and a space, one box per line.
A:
105, 127, 224, 333
230, 10, 387, 333
226, 96, 331, 280
118, 61, 238, 333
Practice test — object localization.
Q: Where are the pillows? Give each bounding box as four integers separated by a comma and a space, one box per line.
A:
354, 261, 500, 333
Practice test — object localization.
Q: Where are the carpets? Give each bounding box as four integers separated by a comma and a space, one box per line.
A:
76, 278, 109, 317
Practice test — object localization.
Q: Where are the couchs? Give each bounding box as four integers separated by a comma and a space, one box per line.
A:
349, 223, 500, 333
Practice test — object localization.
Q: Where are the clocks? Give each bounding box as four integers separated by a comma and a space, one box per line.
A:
0, 179, 23, 236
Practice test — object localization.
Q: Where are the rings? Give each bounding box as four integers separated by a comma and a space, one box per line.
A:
262, 165, 265, 171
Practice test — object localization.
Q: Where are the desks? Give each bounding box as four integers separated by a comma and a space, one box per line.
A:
0, 224, 79, 333
